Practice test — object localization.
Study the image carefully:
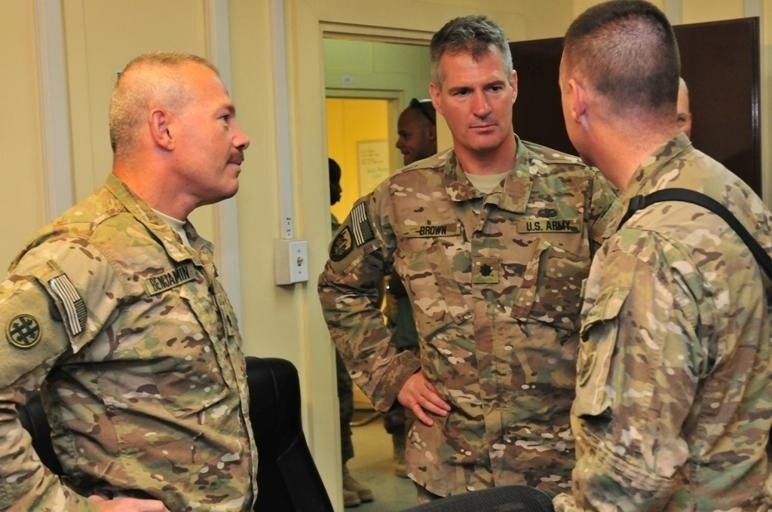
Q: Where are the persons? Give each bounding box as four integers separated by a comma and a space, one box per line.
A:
0, 51, 262, 512
316, 15, 618, 504
379, 95, 437, 480
328, 157, 374, 508
550, 0, 772, 512
